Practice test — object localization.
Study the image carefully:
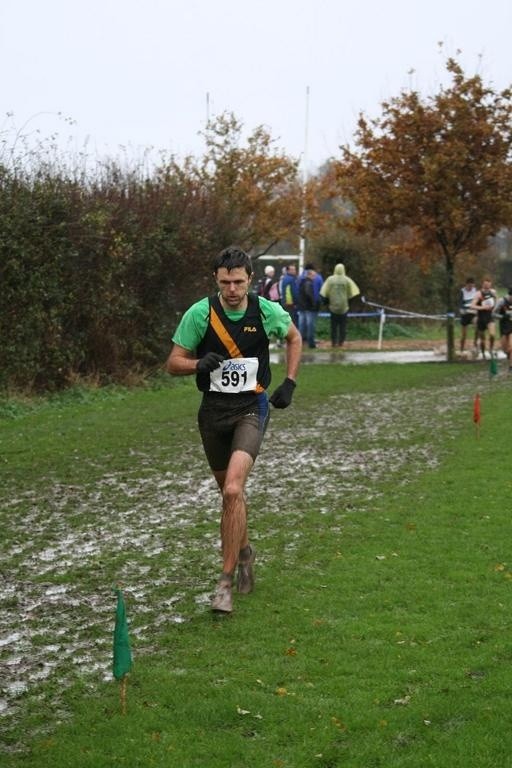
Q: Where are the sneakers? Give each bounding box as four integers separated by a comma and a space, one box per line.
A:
237, 549, 254, 597
212, 572, 233, 614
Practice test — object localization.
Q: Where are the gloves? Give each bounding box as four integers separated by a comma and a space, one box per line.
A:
270, 377, 297, 409
196, 352, 222, 372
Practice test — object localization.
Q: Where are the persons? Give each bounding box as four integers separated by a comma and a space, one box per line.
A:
252, 262, 328, 348
456, 277, 478, 353
490, 287, 512, 373
165, 247, 303, 613
318, 263, 360, 351
469, 276, 497, 361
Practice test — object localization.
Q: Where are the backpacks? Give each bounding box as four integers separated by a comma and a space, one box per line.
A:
330, 287, 347, 314
